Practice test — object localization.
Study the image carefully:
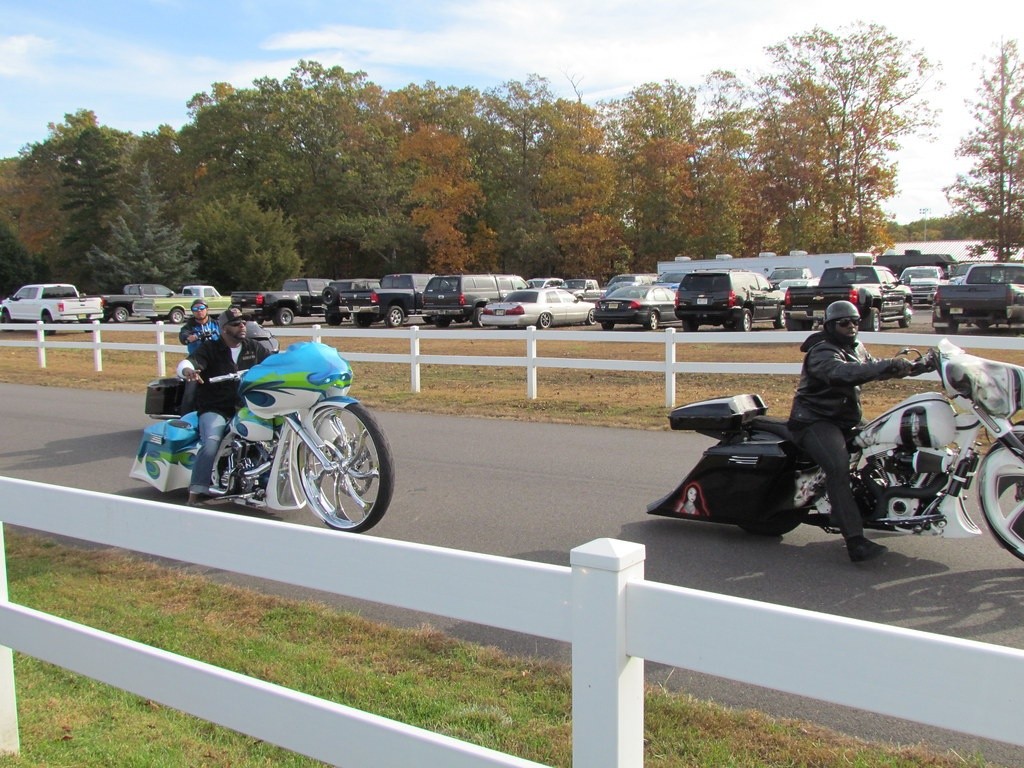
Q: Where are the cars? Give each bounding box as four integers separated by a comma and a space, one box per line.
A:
481, 288, 597, 330
593, 285, 682, 331
599, 282, 638, 299
778, 278, 820, 292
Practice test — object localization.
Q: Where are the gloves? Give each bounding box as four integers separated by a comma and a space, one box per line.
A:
915, 357, 936, 373
892, 357, 913, 373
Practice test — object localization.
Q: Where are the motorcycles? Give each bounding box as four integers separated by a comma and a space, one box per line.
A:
646, 337, 1024, 561
128, 321, 396, 534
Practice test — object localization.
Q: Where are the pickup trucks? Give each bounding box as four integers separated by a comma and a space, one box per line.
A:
1, 283, 104, 336
80, 284, 232, 322
783, 265, 914, 332
932, 263, 1024, 335
231, 278, 335, 326
557, 279, 608, 301
338, 274, 436, 328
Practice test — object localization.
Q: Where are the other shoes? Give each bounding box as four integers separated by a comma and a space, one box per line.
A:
188, 492, 202, 506
848, 540, 888, 562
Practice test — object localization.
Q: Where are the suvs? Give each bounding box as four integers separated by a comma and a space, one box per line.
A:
526, 278, 568, 288
674, 268, 786, 331
322, 278, 380, 326
767, 267, 813, 287
898, 262, 1005, 305
652, 272, 688, 292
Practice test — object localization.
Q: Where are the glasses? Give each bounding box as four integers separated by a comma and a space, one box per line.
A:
191, 305, 205, 312
834, 316, 859, 327
227, 319, 247, 326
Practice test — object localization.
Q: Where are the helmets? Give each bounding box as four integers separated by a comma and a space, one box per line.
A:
190, 299, 208, 308
823, 300, 860, 328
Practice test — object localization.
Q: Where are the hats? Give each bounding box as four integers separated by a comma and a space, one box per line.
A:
219, 308, 251, 327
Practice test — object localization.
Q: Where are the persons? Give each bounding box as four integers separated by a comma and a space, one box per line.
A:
177, 308, 273, 510
179, 294, 222, 355
789, 300, 938, 562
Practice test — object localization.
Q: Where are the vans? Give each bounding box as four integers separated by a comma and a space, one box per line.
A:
607, 273, 659, 284
422, 273, 535, 328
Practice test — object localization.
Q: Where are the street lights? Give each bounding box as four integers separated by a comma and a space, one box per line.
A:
919, 208, 932, 242
